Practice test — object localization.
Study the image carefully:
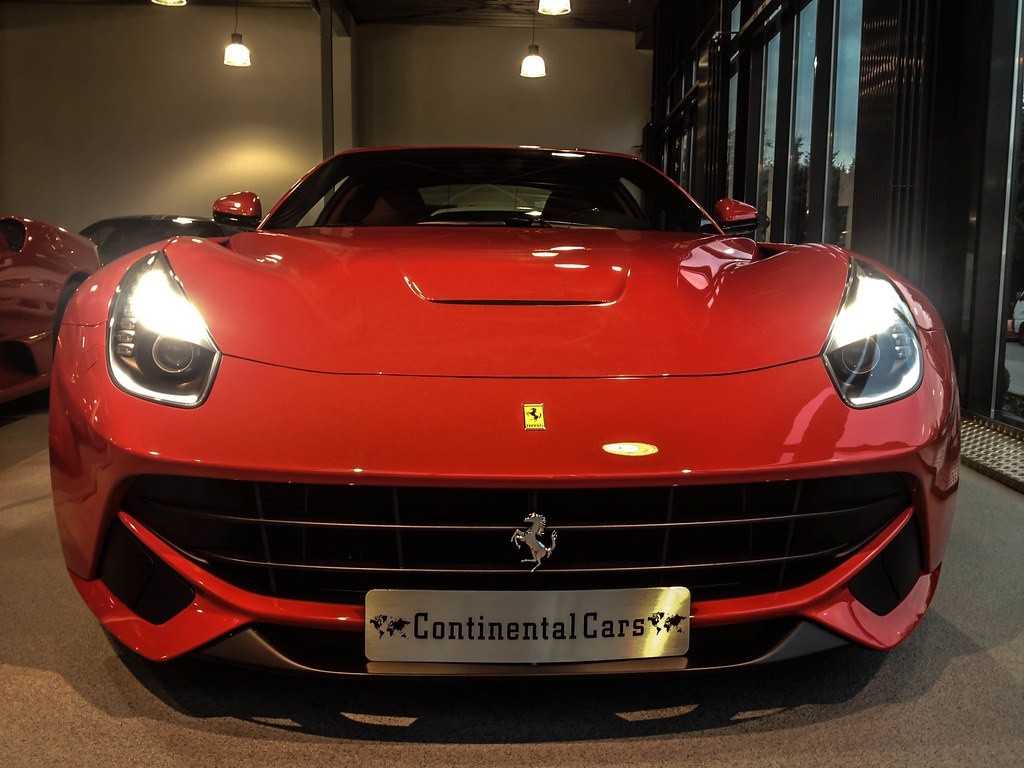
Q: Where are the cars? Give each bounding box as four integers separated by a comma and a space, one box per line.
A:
76, 213, 256, 268
996, 292, 1024, 346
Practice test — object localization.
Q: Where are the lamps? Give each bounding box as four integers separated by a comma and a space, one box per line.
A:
520, 0, 547, 78
537, 0, 572, 16
223, 1, 251, 67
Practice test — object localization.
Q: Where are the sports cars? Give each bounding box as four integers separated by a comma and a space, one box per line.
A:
0, 214, 101, 405
46, 142, 962, 680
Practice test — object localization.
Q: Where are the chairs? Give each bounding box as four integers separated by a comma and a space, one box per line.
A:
537, 187, 618, 222
328, 175, 427, 226
